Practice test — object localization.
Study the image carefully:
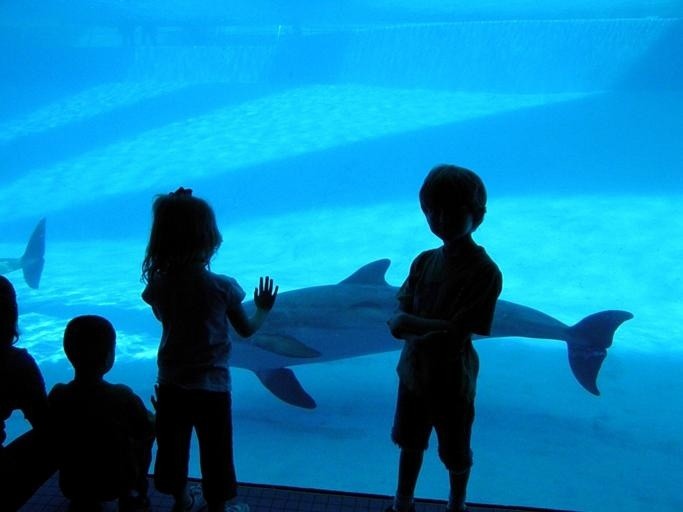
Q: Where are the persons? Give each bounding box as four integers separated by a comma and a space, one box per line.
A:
0, 272, 58, 512
44, 315, 160, 511
139, 187, 279, 512
385, 162, 503, 512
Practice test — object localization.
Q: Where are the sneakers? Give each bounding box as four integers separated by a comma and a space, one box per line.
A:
171, 485, 251, 511
